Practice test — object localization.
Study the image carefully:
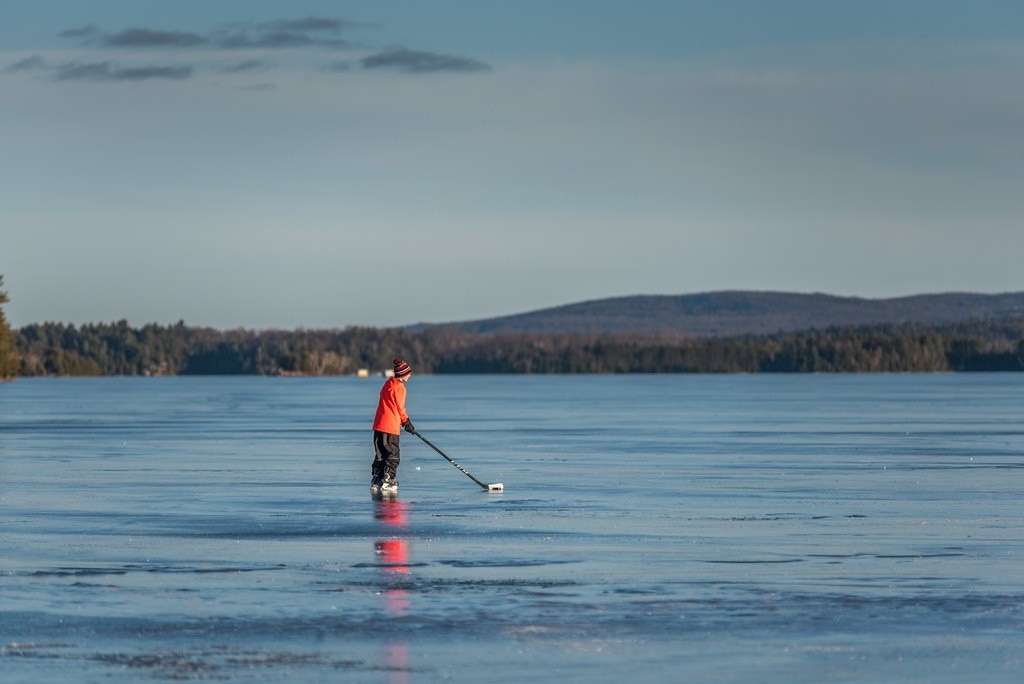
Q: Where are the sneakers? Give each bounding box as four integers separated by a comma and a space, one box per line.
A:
370, 470, 383, 486
381, 473, 399, 490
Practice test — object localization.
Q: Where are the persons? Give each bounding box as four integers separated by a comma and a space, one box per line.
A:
371, 358, 414, 492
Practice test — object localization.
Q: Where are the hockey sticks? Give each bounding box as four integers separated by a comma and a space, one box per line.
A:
414, 430, 504, 491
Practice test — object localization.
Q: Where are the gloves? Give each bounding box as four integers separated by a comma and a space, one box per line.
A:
404, 418, 414, 435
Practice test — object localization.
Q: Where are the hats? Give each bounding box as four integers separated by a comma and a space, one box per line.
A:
393, 359, 411, 377
